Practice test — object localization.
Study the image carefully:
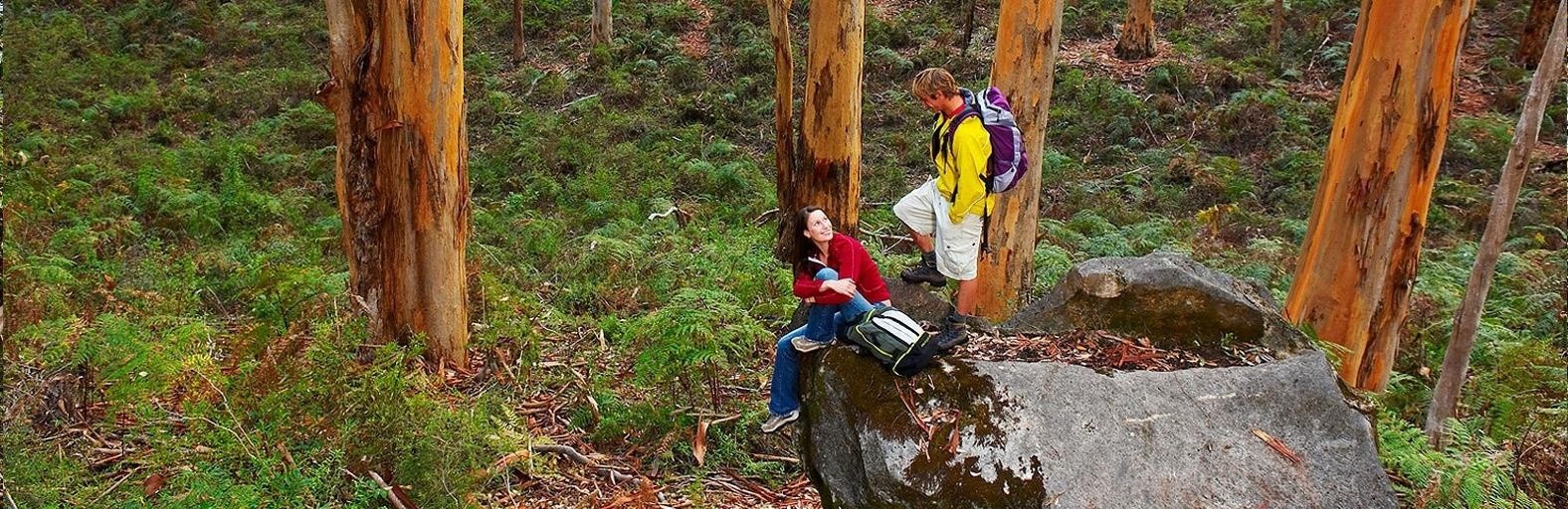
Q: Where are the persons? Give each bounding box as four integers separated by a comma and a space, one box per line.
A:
891, 68, 995, 348
758, 203, 891, 433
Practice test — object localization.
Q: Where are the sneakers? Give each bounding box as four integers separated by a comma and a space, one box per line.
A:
791, 333, 837, 353
761, 410, 800, 434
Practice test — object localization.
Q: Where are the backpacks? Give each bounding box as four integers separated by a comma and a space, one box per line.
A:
844, 306, 939, 378
950, 87, 1028, 192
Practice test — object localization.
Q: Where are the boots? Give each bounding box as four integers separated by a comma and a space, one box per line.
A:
901, 254, 946, 286
933, 316, 969, 350
